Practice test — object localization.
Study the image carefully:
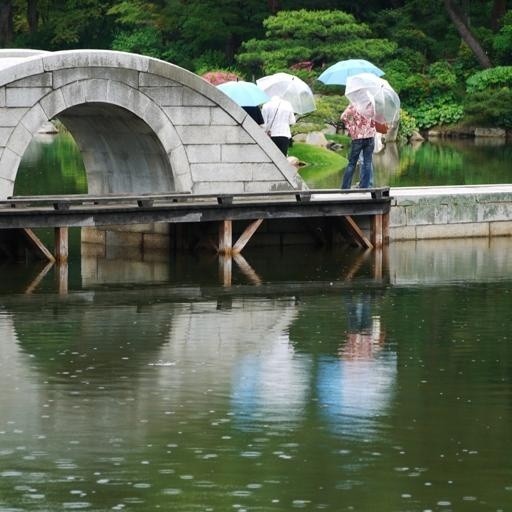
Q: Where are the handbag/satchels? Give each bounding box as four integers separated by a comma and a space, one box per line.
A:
375, 122, 388, 135
264, 129, 272, 139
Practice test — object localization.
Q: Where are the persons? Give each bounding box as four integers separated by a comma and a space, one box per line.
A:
242, 107, 265, 127
354, 113, 388, 189
261, 96, 297, 156
341, 103, 374, 190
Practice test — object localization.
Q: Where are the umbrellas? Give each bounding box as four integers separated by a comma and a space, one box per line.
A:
217, 80, 270, 106
345, 72, 402, 127
256, 72, 317, 115
316, 60, 385, 85
